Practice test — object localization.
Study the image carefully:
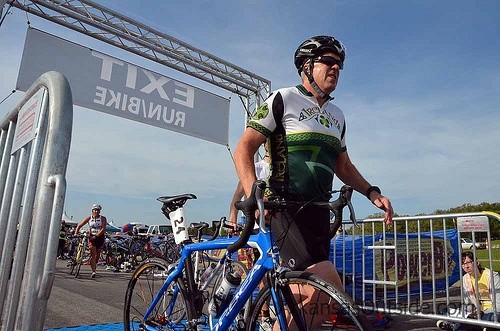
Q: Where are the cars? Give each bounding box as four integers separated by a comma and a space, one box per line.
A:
460, 237, 484, 250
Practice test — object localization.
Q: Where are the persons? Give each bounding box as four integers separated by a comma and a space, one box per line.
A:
56, 220, 75, 260
74, 204, 106, 278
453, 251, 500, 331
233, 35, 394, 331
227, 136, 275, 331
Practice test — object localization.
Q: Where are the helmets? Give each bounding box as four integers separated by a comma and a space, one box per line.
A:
92, 204, 101, 210
295, 35, 346, 70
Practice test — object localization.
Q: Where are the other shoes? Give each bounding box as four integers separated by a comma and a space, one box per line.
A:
259, 320, 273, 331
91, 272, 96, 278
437, 320, 454, 330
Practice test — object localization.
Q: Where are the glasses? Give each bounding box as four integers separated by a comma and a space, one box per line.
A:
310, 56, 344, 70
460, 260, 473, 268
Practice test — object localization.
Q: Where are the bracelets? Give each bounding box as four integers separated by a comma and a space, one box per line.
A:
367, 186, 381, 200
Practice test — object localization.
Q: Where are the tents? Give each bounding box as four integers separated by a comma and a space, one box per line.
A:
61, 211, 123, 234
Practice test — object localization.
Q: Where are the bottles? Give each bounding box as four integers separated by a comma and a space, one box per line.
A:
200, 263, 216, 282
208, 270, 242, 317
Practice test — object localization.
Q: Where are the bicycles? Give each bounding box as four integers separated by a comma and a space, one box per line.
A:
57, 219, 254, 331
123, 180, 375, 331
